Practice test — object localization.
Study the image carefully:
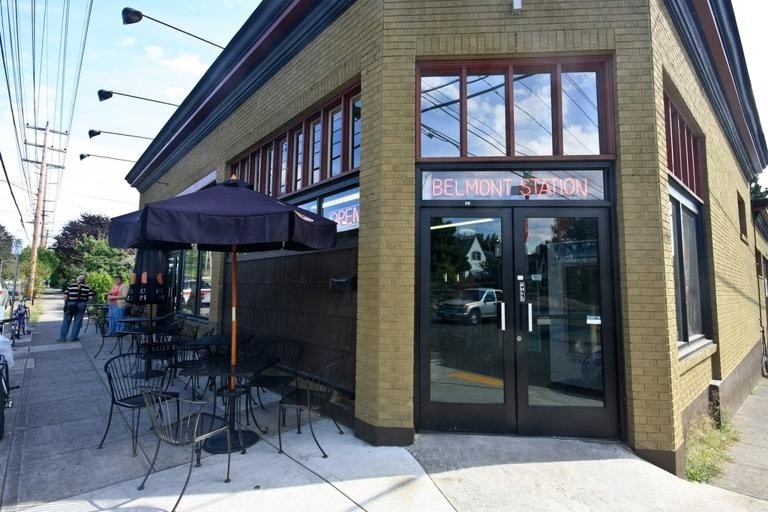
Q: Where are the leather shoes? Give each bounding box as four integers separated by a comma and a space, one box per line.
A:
56, 339, 80, 342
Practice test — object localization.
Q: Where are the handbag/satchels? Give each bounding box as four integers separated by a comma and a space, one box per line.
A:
63, 305, 79, 315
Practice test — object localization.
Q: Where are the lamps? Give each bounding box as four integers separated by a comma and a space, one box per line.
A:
121, 6, 227, 51
79, 87, 180, 164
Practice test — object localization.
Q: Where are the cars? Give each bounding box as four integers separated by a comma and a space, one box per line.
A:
527, 312, 602, 391
174, 280, 211, 308
436, 287, 502, 325
0, 277, 21, 440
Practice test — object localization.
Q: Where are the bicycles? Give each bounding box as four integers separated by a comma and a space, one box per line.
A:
13, 298, 31, 339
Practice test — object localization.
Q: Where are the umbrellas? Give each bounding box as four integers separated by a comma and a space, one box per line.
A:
125, 248, 169, 344
107, 173, 340, 391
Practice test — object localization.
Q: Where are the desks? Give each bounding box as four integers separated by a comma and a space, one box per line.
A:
176, 351, 280, 455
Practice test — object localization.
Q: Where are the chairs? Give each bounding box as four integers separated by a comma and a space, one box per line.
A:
93, 316, 255, 381
136, 388, 235, 512
235, 338, 305, 427
277, 361, 342, 458
97, 352, 181, 456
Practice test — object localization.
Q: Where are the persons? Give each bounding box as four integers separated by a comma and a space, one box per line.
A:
186, 275, 204, 315
56, 274, 93, 342
104, 275, 131, 337
